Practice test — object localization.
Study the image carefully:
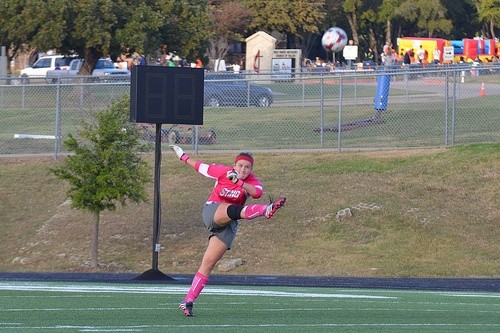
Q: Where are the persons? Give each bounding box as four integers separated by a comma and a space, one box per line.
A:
55, 64, 62, 70
169, 144, 287, 317
105, 42, 500, 75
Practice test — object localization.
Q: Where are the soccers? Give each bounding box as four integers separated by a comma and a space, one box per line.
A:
322, 27, 348, 52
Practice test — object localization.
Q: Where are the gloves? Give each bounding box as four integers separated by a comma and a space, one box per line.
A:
225, 170, 239, 184
169, 144, 186, 160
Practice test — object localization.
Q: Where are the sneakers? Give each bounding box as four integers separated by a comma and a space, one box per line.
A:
178, 300, 195, 317
264, 197, 288, 219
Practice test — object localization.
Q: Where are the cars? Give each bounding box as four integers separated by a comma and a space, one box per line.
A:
204, 74, 272, 108
18, 55, 71, 84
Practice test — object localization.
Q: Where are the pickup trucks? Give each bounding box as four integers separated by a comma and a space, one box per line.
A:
47, 59, 130, 83
